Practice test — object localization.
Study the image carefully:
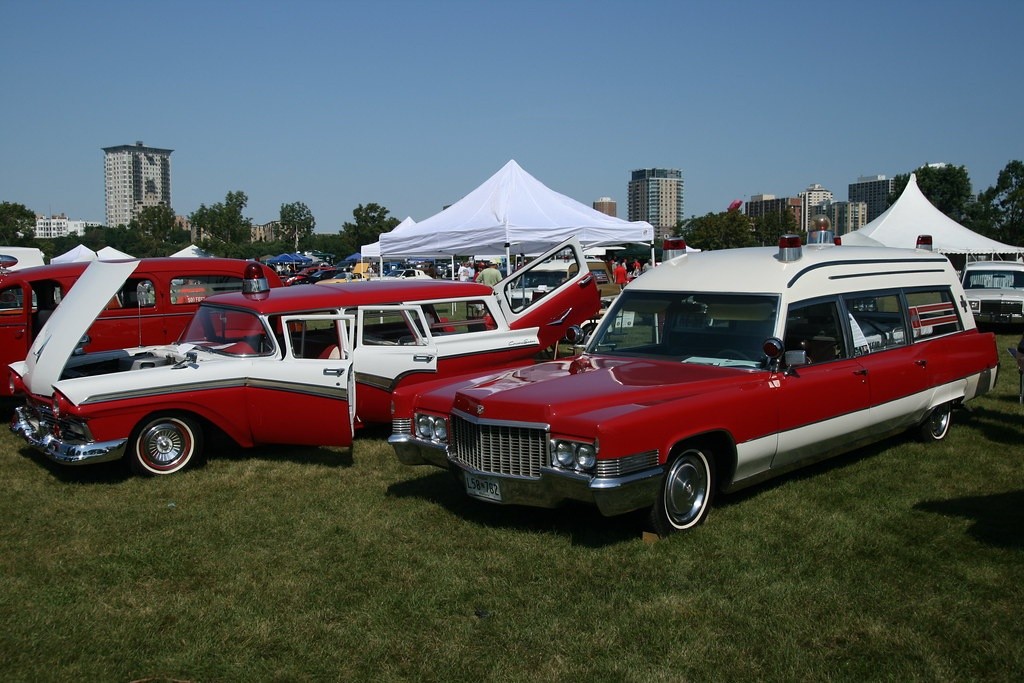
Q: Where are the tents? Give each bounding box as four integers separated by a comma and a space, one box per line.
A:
246, 250, 335, 270
345, 252, 361, 266
169, 244, 218, 258
361, 216, 455, 282
837, 174, 1024, 265
685, 245, 701, 253
474, 255, 508, 264
0, 244, 136, 271
515, 253, 556, 270
379, 159, 659, 344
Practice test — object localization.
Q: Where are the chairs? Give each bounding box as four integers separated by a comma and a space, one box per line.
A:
319, 345, 339, 359
667, 311, 711, 348
782, 316, 821, 354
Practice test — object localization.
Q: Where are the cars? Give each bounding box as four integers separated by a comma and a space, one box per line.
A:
5, 245, 605, 475
954, 258, 1024, 331
265, 253, 463, 287
412, 229, 998, 538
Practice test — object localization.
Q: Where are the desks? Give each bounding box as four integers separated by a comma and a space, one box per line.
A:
509, 287, 552, 311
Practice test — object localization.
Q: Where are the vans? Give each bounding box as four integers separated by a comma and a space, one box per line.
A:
514, 256, 620, 318
0, 258, 287, 431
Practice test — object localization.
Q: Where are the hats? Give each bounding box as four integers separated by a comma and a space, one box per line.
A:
489, 259, 497, 264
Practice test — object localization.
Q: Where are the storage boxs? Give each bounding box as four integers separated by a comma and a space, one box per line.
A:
578, 296, 638, 334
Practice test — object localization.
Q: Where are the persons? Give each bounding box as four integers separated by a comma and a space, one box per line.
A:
517, 260, 527, 270
397, 261, 433, 269
368, 257, 373, 268
269, 262, 292, 275
458, 255, 503, 286
137, 281, 151, 295
608, 258, 651, 293
